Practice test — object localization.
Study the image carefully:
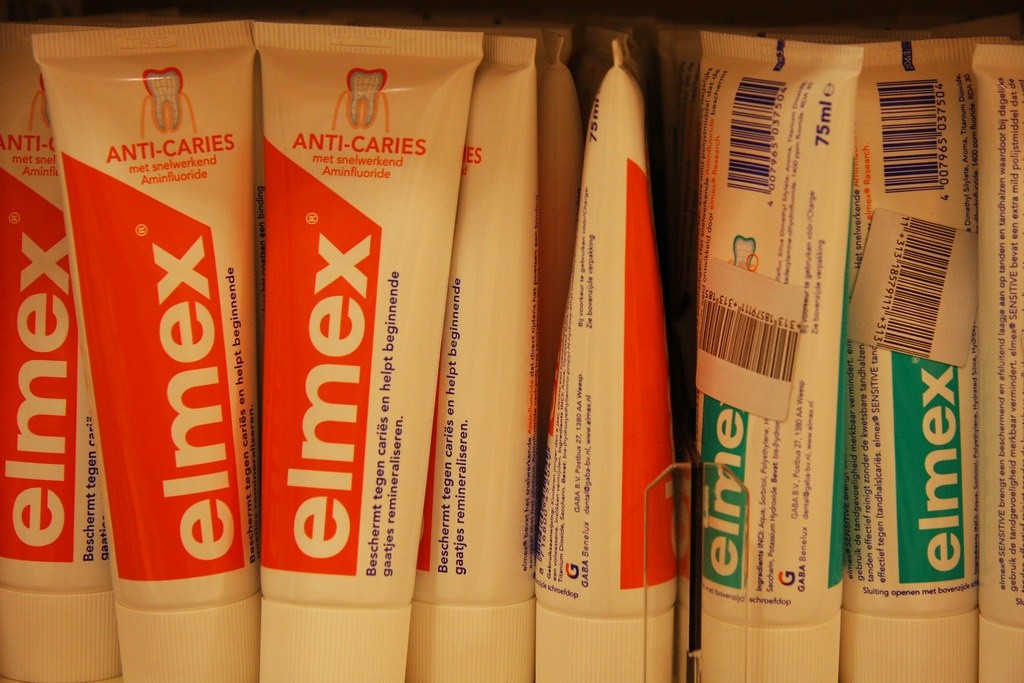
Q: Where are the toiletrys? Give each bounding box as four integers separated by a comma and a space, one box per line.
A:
0, 10, 1024, 683
